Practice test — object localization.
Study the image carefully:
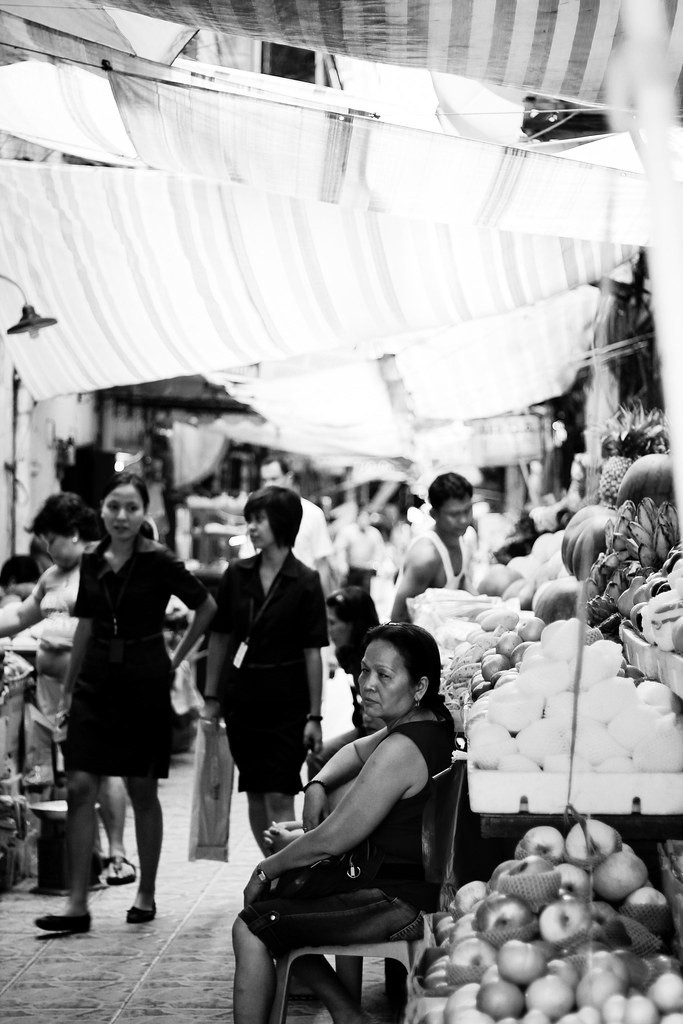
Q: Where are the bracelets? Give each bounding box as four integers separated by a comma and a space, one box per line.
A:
307, 714, 323, 723
257, 862, 272, 884
303, 780, 328, 795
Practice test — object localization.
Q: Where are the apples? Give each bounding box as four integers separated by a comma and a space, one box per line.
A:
466, 617, 683, 777
408, 820, 683, 1024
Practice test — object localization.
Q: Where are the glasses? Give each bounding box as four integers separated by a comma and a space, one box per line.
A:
331, 590, 346, 609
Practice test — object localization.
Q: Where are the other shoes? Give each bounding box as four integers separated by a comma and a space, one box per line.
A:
287, 983, 321, 1001
36, 912, 90, 932
126, 897, 155, 923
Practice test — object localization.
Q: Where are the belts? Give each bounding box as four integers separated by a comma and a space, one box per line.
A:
97, 633, 162, 645
230, 656, 305, 668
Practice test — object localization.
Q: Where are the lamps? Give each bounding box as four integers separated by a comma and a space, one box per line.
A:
0, 274, 57, 338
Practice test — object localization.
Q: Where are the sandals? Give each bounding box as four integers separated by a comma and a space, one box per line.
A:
107, 855, 136, 884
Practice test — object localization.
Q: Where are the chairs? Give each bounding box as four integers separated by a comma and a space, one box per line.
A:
272, 750, 471, 1024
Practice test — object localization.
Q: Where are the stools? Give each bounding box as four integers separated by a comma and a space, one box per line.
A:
24, 799, 103, 894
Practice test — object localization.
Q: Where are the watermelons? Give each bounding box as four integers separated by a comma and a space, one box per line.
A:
533, 516, 620, 623
615, 454, 673, 515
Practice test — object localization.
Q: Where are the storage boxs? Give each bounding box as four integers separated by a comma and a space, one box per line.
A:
463, 619, 683, 816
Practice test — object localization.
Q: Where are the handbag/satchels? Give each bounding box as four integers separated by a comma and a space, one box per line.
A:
188, 719, 234, 863
272, 838, 385, 902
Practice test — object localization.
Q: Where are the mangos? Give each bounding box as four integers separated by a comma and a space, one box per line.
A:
446, 607, 520, 702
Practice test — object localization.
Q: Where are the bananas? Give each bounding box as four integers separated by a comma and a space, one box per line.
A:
580, 497, 680, 625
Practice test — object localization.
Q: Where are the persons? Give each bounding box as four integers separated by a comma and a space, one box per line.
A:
240, 456, 331, 601
308, 585, 382, 781
332, 472, 474, 625
231, 621, 457, 1024
0, 456, 216, 931
203, 487, 329, 857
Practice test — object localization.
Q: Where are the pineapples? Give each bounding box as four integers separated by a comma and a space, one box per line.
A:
598, 401, 654, 504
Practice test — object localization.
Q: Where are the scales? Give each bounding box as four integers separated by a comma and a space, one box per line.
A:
28, 799, 101, 894
